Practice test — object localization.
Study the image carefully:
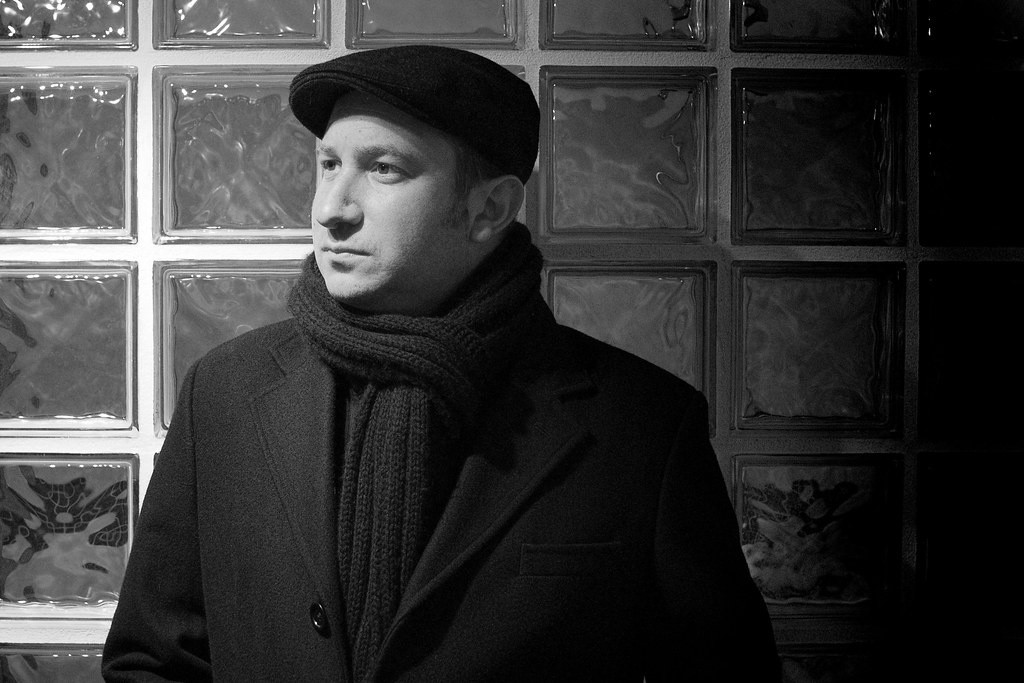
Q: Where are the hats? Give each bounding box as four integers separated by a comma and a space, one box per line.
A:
289, 45, 541, 186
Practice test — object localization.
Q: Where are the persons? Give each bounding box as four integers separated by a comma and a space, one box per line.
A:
66, 44, 785, 683
